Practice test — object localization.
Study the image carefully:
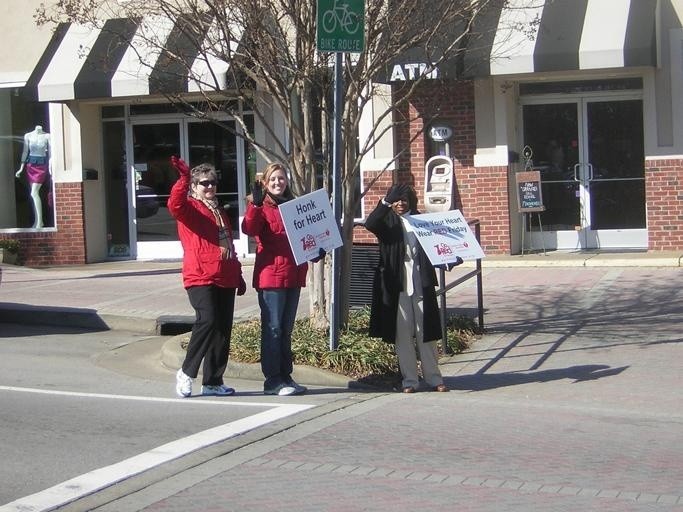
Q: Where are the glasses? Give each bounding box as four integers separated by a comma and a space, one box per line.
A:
197, 180, 218, 186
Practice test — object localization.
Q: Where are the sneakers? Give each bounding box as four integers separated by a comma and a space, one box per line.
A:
263, 381, 307, 395
202, 383, 235, 395
404, 386, 413, 393
176, 368, 194, 398
436, 385, 448, 392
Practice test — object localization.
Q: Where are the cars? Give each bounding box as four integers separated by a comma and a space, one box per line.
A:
136, 185, 160, 218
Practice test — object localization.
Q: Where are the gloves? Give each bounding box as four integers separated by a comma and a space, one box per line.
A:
448, 256, 463, 267
237, 278, 246, 295
311, 248, 326, 262
385, 182, 409, 204
172, 156, 191, 175
250, 181, 267, 208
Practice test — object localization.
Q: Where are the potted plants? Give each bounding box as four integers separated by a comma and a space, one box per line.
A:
0, 239, 20, 265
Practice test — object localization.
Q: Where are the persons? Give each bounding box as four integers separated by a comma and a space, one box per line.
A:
16, 125, 52, 228
241, 162, 326, 396
364, 182, 464, 393
167, 156, 246, 398
